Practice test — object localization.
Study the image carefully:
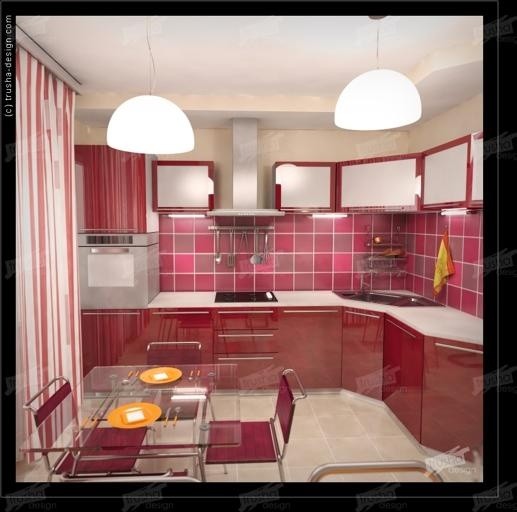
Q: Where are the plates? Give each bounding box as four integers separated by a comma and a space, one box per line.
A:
139, 367, 184, 385
106, 402, 163, 430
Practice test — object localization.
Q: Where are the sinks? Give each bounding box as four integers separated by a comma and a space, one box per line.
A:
331, 290, 444, 306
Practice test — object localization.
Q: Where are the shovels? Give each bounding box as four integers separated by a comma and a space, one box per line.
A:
228, 227, 234, 267
250, 227, 263, 265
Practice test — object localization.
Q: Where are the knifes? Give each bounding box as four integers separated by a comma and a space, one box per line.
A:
131, 370, 139, 385
163, 407, 171, 427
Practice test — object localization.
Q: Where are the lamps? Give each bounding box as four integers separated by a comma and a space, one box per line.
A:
104, 15, 196, 157
332, 12, 423, 130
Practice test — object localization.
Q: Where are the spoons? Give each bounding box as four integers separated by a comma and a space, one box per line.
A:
173, 405, 184, 428
120, 368, 132, 385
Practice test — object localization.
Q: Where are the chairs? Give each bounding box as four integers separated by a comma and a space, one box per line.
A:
19, 340, 309, 480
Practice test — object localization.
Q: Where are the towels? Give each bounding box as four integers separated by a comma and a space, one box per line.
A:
431, 230, 456, 296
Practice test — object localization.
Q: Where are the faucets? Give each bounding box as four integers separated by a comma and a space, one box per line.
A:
359, 274, 369, 291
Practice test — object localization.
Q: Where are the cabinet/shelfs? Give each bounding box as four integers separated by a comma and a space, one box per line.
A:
76, 144, 159, 233
338, 152, 422, 212
213, 306, 279, 392
384, 313, 423, 443
421, 337, 484, 466
83, 310, 148, 393
357, 224, 410, 278
421, 134, 472, 211
342, 306, 382, 404
152, 160, 216, 214
148, 308, 212, 392
271, 160, 336, 213
469, 132, 485, 209
279, 309, 341, 390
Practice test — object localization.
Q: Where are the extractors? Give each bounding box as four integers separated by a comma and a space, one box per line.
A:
214, 291, 278, 302
